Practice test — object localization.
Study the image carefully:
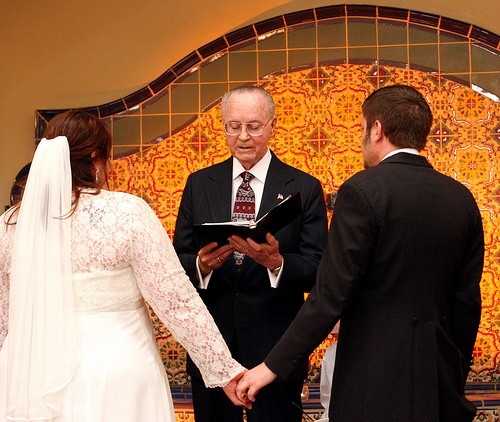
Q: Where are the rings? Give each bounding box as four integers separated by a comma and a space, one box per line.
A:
216, 257, 222, 263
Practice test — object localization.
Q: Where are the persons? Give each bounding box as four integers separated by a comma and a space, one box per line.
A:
0, 112, 253, 422
171, 87, 327, 422
235, 84, 484, 422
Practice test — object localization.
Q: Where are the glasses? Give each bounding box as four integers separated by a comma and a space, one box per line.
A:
223, 118, 272, 137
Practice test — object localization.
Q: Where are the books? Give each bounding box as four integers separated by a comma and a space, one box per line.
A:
192, 191, 303, 255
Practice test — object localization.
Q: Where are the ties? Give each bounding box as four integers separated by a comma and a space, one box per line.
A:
230, 171, 255, 274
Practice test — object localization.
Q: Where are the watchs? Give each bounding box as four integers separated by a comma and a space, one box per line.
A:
271, 265, 281, 275
